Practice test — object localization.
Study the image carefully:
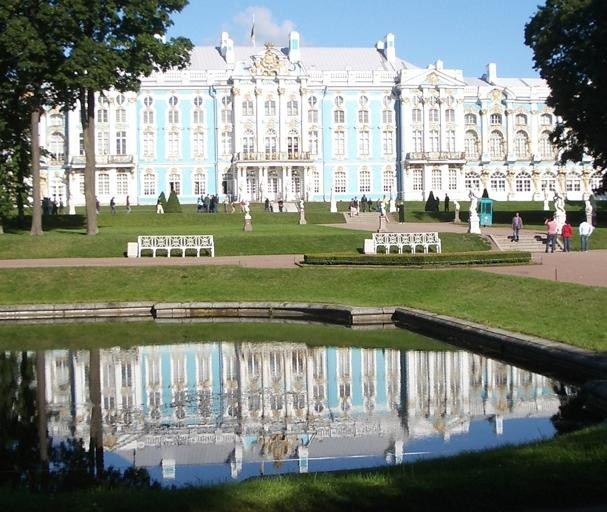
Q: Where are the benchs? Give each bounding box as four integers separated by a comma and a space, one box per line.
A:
137, 234, 214, 258
372, 232, 441, 254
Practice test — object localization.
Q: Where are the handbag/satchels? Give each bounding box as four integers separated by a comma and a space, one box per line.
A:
516, 225, 521, 231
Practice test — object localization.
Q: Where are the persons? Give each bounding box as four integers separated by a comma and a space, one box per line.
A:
67, 190, 74, 202
445, 193, 449, 213
390, 190, 397, 201
95, 196, 100, 216
245, 200, 251, 216
156, 202, 164, 214
299, 199, 304, 209
560, 220, 572, 252
350, 194, 390, 212
544, 216, 557, 253
126, 195, 131, 214
512, 212, 523, 241
585, 200, 593, 214
395, 201, 404, 222
239, 199, 250, 214
468, 190, 477, 216
110, 196, 117, 216
265, 197, 283, 212
196, 193, 236, 215
579, 218, 595, 252
51, 197, 64, 216
554, 188, 566, 214
454, 201, 460, 210
